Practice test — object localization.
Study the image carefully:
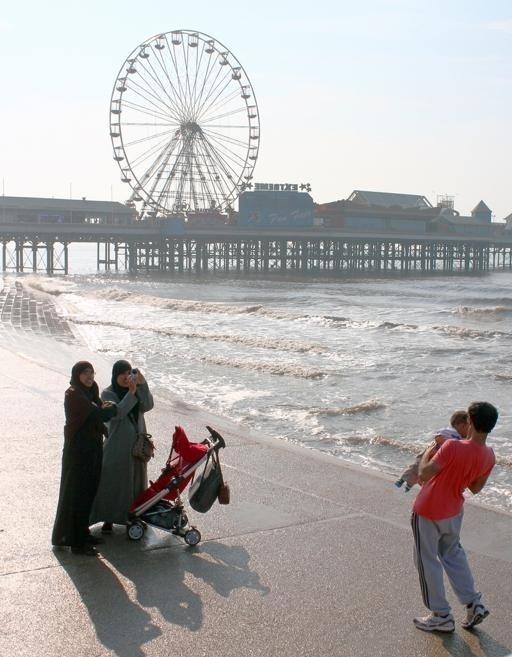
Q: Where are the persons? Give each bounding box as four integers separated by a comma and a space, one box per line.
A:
87, 359, 156, 535
394, 409, 470, 493
409, 400, 500, 634
50, 360, 119, 555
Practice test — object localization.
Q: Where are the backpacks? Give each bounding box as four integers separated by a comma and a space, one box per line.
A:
188, 442, 220, 513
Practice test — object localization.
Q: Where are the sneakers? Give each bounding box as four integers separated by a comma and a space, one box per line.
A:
102, 522, 112, 534
460, 600, 491, 630
86, 535, 105, 544
413, 614, 456, 633
71, 542, 97, 555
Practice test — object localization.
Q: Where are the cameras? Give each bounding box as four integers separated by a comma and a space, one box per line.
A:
129, 368, 139, 378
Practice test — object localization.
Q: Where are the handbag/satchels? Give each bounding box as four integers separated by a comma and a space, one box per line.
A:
218, 484, 229, 505
132, 433, 156, 462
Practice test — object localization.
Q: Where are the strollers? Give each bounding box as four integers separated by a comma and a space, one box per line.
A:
124, 422, 226, 547
